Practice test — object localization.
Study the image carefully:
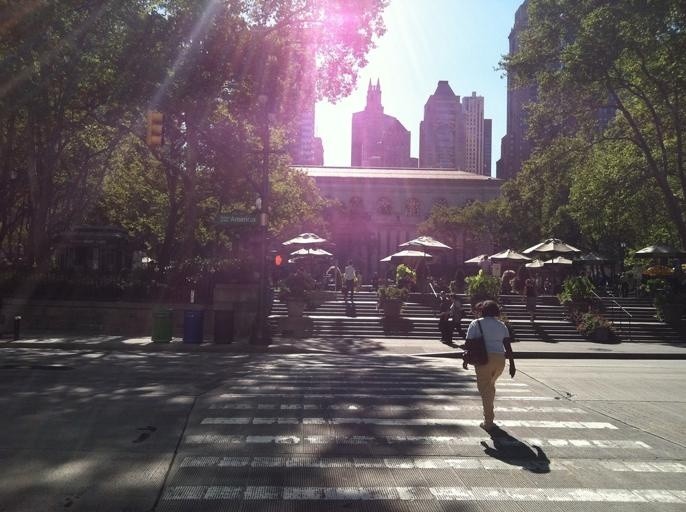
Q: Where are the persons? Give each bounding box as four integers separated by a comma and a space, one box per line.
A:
438, 291, 465, 346
372, 271, 379, 293
481, 256, 491, 278
461, 300, 517, 433
521, 277, 537, 322
343, 259, 357, 304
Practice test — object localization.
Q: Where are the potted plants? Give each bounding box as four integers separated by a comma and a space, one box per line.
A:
464, 269, 505, 313
277, 268, 325, 318
376, 262, 418, 316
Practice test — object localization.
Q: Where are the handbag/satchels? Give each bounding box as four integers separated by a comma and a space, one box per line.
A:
469, 337, 488, 365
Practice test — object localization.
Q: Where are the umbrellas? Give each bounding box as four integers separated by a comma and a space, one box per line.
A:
378, 234, 452, 265
281, 232, 335, 265
465, 237, 616, 273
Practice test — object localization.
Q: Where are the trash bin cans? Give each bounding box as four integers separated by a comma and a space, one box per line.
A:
151, 309, 174, 343
214, 310, 234, 344
184, 310, 205, 343
